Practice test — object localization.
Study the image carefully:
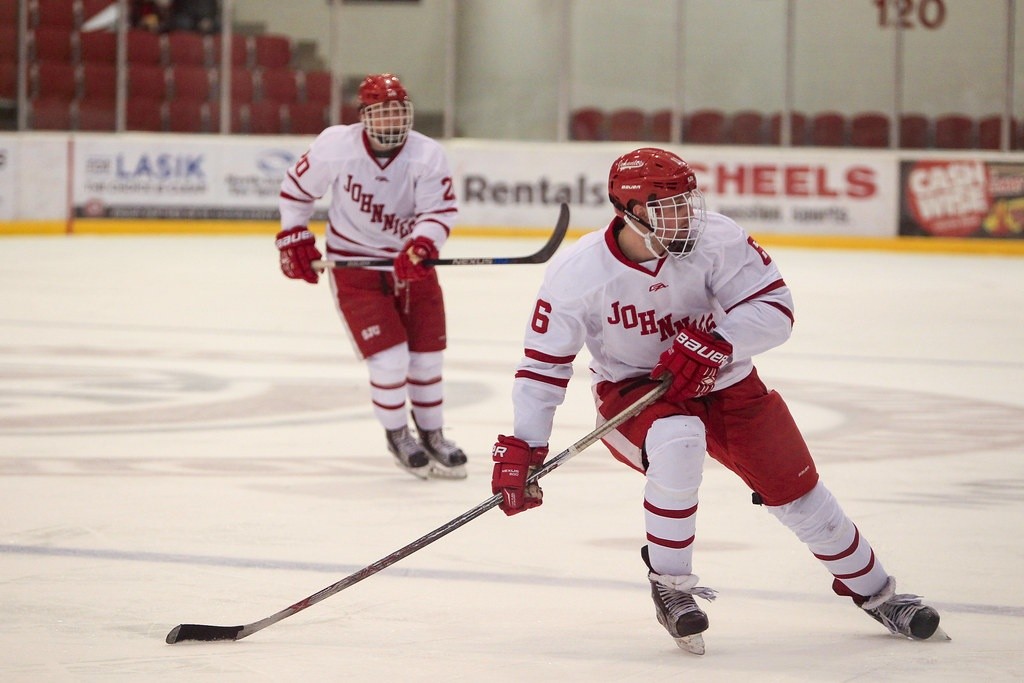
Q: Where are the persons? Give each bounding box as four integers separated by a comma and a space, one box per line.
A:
131, 0, 222, 34
491, 149, 952, 655
275, 74, 469, 480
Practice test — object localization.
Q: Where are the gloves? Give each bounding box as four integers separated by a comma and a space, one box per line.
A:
648, 328, 733, 403
491, 434, 549, 516
393, 236, 439, 282
276, 225, 324, 284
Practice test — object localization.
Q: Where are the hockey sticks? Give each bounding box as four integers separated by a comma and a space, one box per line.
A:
311, 202, 571, 270
164, 379, 670, 646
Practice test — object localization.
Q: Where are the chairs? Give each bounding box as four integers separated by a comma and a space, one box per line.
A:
570, 109, 1024, 152
0, 0, 360, 135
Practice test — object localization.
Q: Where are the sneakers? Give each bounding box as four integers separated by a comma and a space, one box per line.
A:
386, 426, 432, 479
411, 409, 468, 478
640, 544, 719, 655
833, 577, 952, 643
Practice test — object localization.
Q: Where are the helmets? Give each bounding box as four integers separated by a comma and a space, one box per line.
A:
607, 147, 707, 260
358, 73, 414, 149
